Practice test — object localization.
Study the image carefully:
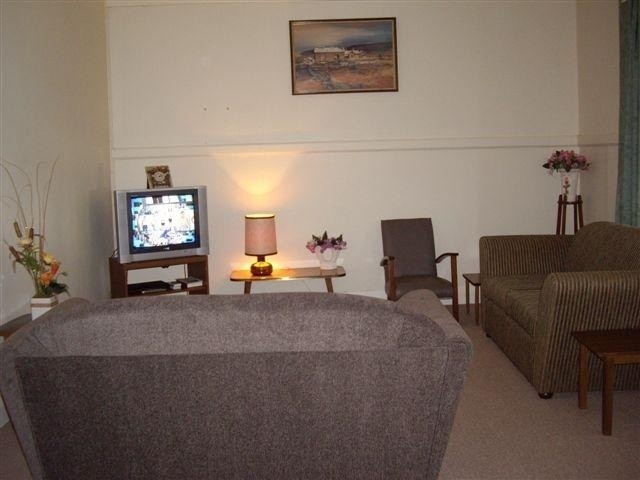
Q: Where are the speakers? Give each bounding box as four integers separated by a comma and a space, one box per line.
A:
116, 185, 209, 265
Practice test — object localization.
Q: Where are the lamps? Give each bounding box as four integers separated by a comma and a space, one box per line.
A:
244, 214, 277, 275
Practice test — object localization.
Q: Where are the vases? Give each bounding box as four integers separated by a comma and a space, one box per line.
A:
314, 245, 342, 270
559, 169, 579, 202
31, 285, 70, 320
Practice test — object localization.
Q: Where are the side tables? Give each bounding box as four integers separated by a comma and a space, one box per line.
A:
462, 273, 480, 323
0, 314, 31, 340
571, 329, 640, 436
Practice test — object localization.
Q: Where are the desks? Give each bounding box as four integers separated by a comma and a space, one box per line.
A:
230, 267, 346, 294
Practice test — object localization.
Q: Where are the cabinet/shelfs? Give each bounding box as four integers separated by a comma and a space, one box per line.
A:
108, 256, 209, 298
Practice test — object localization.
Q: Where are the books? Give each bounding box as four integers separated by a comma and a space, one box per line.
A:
176, 276, 203, 288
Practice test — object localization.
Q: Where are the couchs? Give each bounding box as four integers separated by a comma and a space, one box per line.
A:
0, 288, 474, 480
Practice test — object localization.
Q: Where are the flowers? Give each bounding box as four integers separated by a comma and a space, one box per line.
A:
306, 231, 346, 253
541, 149, 592, 173
0, 151, 70, 296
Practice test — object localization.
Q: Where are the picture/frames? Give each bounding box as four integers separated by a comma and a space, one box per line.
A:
289, 18, 398, 94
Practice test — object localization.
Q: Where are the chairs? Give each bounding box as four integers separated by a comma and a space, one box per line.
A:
380, 218, 458, 324
478, 221, 639, 399
